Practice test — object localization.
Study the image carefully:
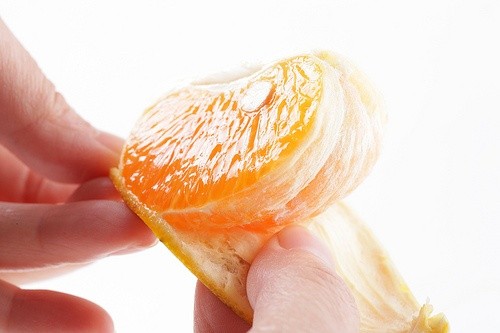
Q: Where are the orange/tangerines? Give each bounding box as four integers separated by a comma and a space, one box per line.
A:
118, 50, 384, 234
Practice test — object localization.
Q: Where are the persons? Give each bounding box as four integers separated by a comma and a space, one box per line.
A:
0, 15, 366, 333
